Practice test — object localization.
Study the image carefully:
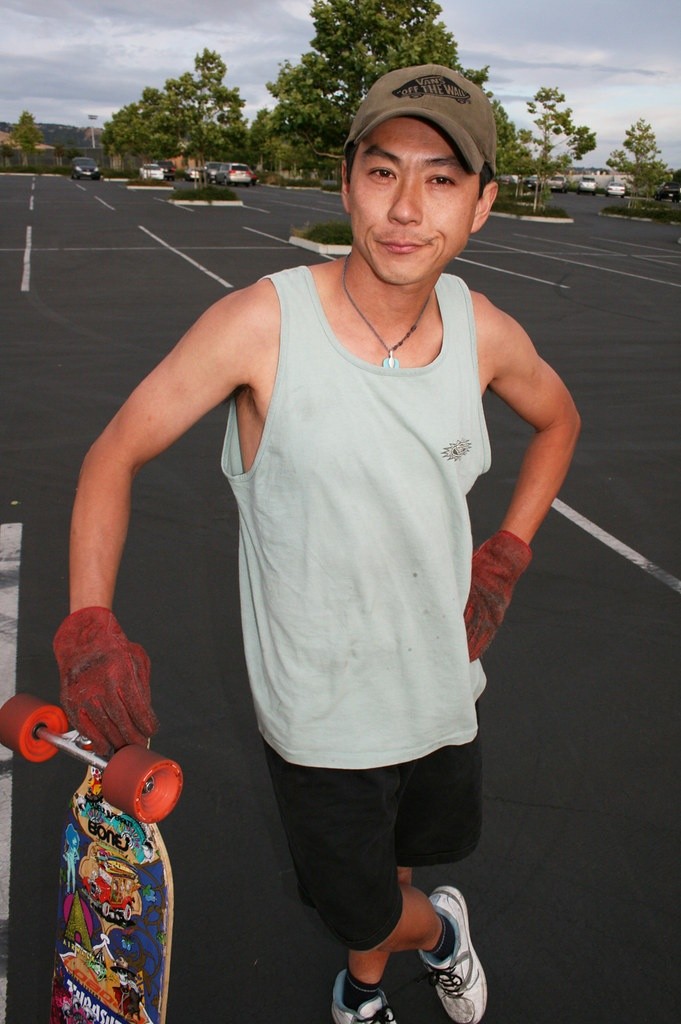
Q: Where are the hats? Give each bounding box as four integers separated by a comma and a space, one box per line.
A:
344, 64, 498, 177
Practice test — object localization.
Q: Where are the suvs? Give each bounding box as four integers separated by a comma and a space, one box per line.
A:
70, 158, 100, 180
655, 183, 681, 203
151, 160, 176, 180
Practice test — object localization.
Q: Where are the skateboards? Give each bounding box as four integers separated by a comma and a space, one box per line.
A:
2, 692, 184, 1024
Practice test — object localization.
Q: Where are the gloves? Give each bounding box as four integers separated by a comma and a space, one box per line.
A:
465, 530, 534, 663
52, 608, 159, 751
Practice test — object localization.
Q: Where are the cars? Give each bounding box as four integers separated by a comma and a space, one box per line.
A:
606, 181, 626, 198
547, 175, 569, 193
577, 176, 597, 195
185, 167, 201, 181
202, 162, 223, 183
495, 174, 516, 185
139, 164, 164, 181
213, 163, 252, 186
522, 176, 542, 191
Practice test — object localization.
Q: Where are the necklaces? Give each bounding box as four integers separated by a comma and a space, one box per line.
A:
342, 253, 429, 369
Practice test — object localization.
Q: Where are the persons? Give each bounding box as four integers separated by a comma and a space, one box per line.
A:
51, 62, 584, 1022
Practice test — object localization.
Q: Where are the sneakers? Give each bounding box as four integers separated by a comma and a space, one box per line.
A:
330, 970, 398, 1024
418, 885, 488, 1024
